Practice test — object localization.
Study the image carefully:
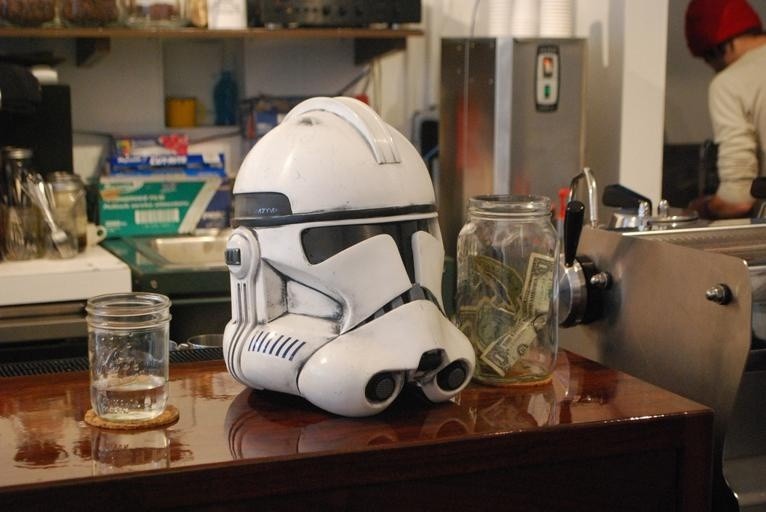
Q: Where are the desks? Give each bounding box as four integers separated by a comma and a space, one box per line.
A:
0, 336, 713, 510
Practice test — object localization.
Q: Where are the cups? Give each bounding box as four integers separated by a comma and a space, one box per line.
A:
51, 181, 88, 259
166, 95, 209, 128
84, 291, 174, 423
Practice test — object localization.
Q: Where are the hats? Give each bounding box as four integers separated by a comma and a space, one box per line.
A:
685, 0, 761, 57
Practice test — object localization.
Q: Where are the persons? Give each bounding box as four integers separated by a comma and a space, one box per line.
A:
684, 1, 765, 219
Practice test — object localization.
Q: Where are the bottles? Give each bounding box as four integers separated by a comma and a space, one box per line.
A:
454, 192, 561, 386
0, 148, 46, 262
214, 68, 239, 126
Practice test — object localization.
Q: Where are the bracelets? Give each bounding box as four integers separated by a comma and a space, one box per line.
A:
704, 194, 713, 216
